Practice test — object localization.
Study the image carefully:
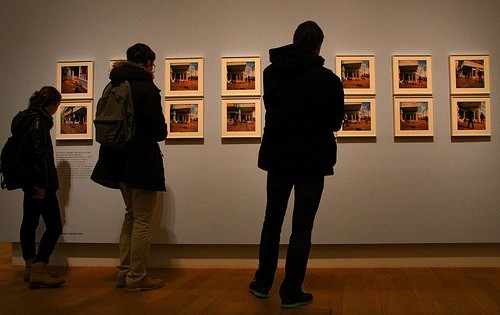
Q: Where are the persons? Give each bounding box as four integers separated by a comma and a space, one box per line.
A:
249, 20, 344, 306
11, 85, 65, 288
343, 113, 348, 126
90, 43, 168, 291
480, 113, 485, 123
466, 108, 474, 129
477, 70, 482, 83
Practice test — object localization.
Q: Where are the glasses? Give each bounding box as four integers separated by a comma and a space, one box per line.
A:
152, 63, 155, 68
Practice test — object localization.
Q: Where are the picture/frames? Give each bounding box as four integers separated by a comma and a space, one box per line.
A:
336, 55, 375, 95
449, 54, 490, 95
164, 57, 204, 98
164, 98, 204, 139
57, 60, 94, 100
393, 55, 432, 95
393, 96, 434, 136
221, 97, 262, 139
222, 56, 261, 96
56, 100, 94, 140
336, 96, 376, 137
450, 96, 491, 136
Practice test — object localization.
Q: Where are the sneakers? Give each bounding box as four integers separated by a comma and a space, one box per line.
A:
116, 267, 130, 286
125, 272, 164, 292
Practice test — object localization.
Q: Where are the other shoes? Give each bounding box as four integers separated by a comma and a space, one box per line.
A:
281, 291, 313, 308
248, 281, 269, 297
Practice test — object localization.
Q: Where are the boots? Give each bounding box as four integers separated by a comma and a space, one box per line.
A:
24, 259, 57, 282
29, 262, 65, 289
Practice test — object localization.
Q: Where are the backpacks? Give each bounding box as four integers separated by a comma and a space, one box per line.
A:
0, 136, 31, 190
93, 80, 135, 148
